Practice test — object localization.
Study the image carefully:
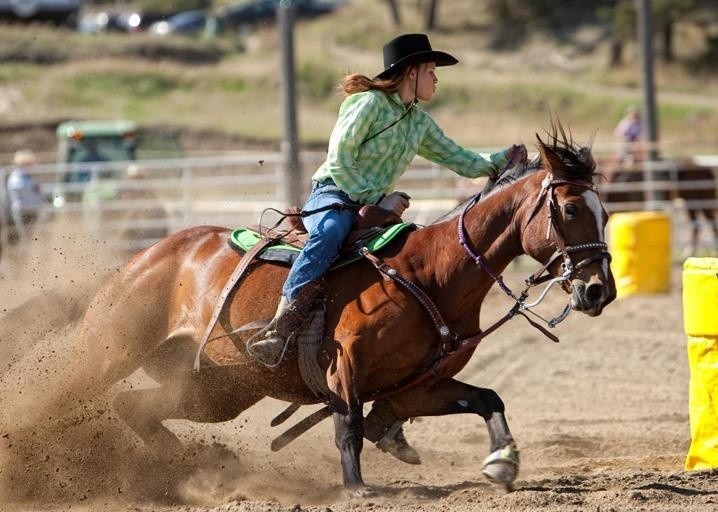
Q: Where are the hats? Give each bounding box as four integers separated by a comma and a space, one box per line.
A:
376, 34, 458, 79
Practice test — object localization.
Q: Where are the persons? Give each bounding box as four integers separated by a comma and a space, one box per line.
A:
609, 103, 641, 201
247, 35, 527, 369
6, 135, 169, 263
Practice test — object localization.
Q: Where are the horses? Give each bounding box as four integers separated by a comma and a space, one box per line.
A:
594, 152, 717, 257
0, 102, 618, 500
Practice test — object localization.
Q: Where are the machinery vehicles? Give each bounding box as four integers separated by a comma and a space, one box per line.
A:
51, 119, 142, 213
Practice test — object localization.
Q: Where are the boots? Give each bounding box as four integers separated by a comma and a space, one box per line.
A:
251, 295, 289, 365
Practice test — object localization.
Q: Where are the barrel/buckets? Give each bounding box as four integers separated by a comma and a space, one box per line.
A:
604, 210, 674, 300
